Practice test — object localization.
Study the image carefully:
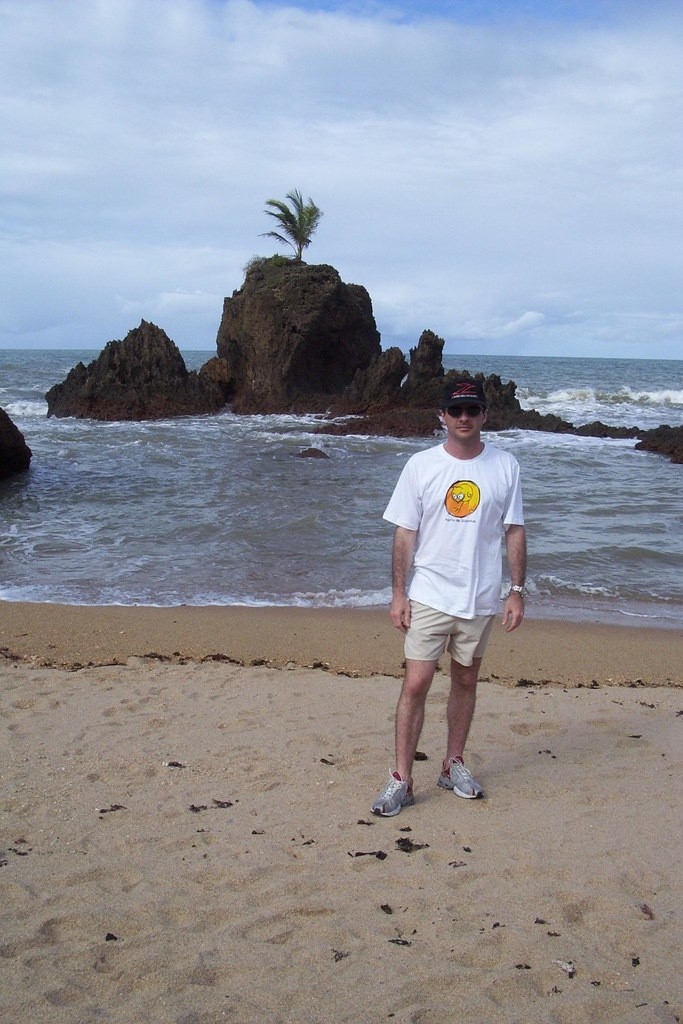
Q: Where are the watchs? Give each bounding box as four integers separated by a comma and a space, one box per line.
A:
509, 585, 528, 598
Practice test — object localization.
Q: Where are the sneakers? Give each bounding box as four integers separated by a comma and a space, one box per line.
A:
437, 755, 483, 798
370, 767, 414, 816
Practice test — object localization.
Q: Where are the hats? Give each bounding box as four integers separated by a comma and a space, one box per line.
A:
441, 379, 487, 408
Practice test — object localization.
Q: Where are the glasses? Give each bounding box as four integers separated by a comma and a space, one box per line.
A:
445, 405, 485, 417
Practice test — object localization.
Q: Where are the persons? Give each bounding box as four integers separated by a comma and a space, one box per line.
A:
371, 377, 529, 815
